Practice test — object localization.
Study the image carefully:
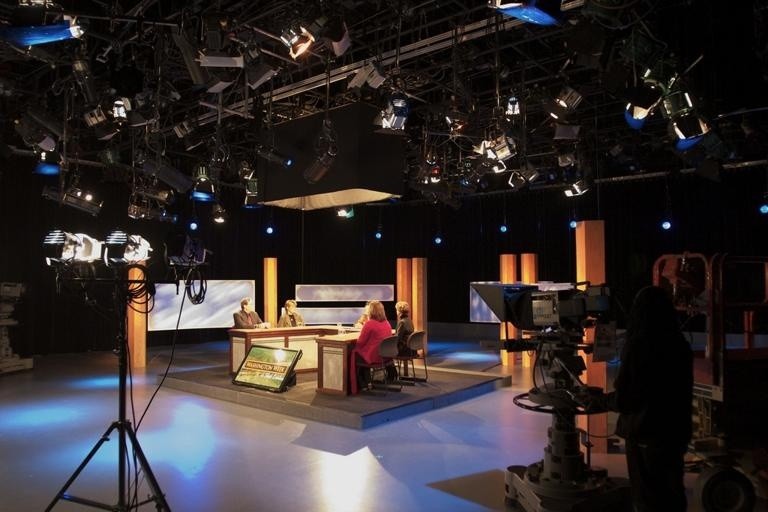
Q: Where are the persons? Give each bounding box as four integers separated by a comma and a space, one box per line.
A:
570, 282, 697, 512
382, 300, 417, 385
346, 298, 395, 396
276, 298, 305, 328
232, 297, 271, 330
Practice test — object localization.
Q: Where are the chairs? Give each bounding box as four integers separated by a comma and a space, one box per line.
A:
359, 329, 429, 396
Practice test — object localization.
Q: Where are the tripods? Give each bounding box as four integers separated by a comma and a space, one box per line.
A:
41, 296, 175, 512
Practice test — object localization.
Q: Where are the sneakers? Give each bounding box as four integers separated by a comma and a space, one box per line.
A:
382, 363, 400, 383
359, 382, 374, 392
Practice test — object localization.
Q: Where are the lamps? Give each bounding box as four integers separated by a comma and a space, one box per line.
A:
12, 0, 711, 220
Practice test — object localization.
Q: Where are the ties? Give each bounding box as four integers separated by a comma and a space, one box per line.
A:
247, 314, 253, 325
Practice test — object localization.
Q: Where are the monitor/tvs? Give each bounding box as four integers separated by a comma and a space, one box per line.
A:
232, 344, 303, 392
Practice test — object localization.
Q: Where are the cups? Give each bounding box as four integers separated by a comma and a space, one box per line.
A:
335, 319, 346, 337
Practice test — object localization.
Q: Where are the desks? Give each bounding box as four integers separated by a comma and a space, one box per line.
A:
228, 325, 396, 395
